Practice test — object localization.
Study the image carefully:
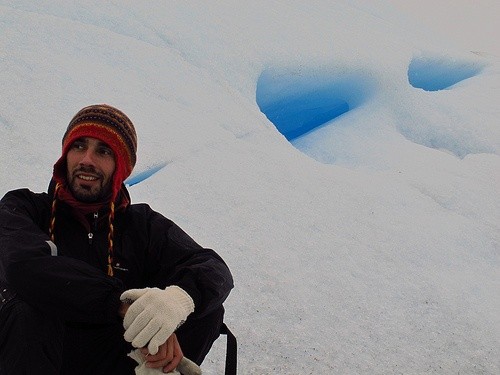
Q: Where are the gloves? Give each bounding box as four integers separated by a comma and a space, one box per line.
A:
121, 285, 195, 355
127, 348, 202, 375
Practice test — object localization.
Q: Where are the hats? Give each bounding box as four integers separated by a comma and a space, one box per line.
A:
48, 105, 137, 276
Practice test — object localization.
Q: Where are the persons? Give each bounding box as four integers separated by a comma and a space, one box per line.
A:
0, 103, 234, 374
